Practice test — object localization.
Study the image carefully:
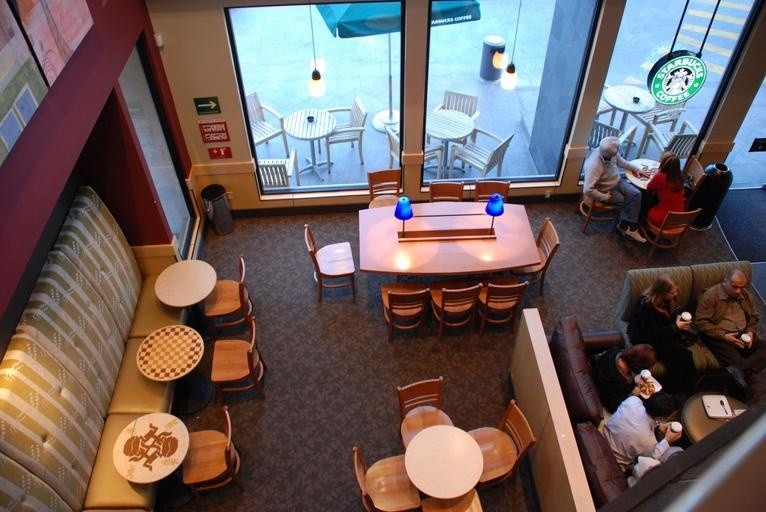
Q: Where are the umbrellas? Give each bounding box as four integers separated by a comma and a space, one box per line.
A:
313, 0, 481, 134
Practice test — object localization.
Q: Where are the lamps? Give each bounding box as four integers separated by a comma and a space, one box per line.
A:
493, 46, 508, 69
307, 3, 328, 100
502, 1, 526, 90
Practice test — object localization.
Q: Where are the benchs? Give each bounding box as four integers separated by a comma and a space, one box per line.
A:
0, 187, 185, 508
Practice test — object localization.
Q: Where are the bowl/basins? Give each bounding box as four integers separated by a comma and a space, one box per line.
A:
633, 97, 640, 104
306, 116, 314, 123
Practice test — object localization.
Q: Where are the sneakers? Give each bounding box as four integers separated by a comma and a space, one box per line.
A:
616, 223, 647, 243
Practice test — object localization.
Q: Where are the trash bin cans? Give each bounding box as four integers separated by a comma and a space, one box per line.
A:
201, 183, 235, 236
477, 35, 506, 84
686, 162, 733, 231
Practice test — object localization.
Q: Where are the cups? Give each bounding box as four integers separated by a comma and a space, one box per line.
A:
680, 312, 692, 322
640, 369, 651, 382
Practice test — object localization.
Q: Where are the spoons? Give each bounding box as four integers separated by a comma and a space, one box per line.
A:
719, 400, 729, 416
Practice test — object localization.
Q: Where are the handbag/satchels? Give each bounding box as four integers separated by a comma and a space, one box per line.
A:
683, 175, 693, 199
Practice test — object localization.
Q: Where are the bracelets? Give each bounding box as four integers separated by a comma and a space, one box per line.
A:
656, 420, 661, 428
635, 168, 641, 172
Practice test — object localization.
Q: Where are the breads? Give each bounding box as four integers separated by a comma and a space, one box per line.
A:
639, 382, 655, 396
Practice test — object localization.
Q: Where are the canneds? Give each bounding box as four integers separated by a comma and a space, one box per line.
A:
679, 311, 692, 322
640, 369, 651, 380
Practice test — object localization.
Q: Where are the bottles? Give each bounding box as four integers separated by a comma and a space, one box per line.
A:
727, 366, 745, 385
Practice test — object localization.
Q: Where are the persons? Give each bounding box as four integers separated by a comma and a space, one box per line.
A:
582, 137, 647, 244
665, 69, 689, 94
628, 274, 692, 382
647, 150, 687, 230
591, 343, 658, 416
603, 392, 684, 473
695, 269, 766, 390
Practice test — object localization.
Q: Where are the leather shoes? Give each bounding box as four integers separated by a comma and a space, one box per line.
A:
726, 366, 748, 389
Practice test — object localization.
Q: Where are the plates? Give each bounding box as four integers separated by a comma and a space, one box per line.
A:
634, 375, 664, 400
702, 394, 733, 419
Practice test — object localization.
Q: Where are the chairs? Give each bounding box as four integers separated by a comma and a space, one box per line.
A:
587, 81, 698, 169
244, 88, 515, 190
